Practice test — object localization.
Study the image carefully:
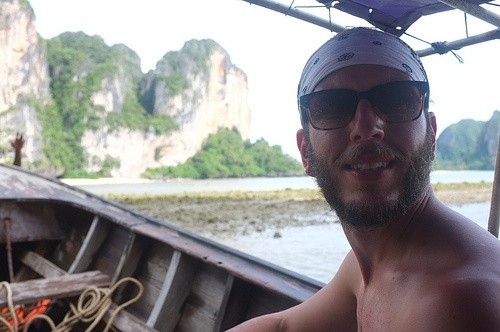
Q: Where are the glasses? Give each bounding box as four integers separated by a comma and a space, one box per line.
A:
299, 81, 430, 131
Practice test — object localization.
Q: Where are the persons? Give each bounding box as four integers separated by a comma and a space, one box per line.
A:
221, 27, 498, 331
8, 129, 28, 168
